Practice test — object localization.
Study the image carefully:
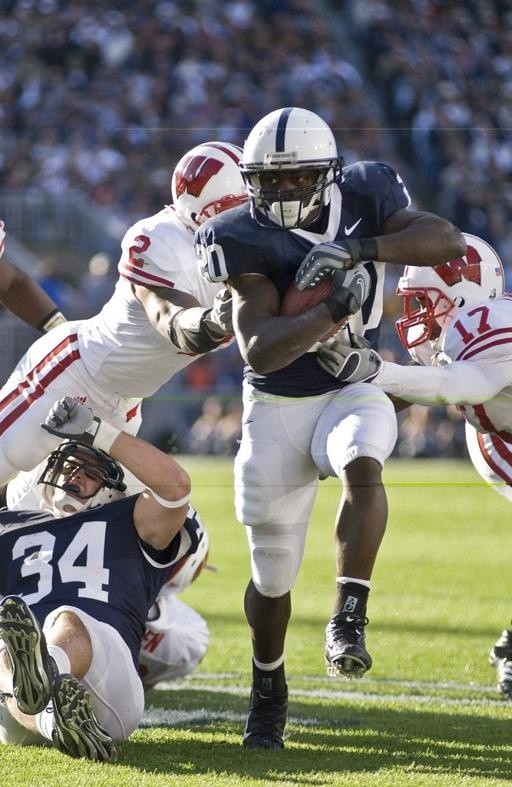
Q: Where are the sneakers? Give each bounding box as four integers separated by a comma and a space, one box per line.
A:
322, 612, 376, 681
490, 627, 512, 698
48, 672, 120, 765
240, 677, 290, 753
0, 594, 52, 717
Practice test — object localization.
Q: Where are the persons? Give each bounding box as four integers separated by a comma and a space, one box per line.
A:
194, 106, 468, 750
1, 1, 512, 461
0, 394, 201, 764
314, 228, 512, 705
0, 503, 212, 745
0, 137, 250, 513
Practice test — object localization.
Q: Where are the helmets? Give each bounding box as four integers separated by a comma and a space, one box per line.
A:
34, 438, 128, 514
398, 230, 508, 336
170, 140, 252, 235
233, 104, 337, 211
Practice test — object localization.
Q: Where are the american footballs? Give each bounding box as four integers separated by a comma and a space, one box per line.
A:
282, 277, 353, 342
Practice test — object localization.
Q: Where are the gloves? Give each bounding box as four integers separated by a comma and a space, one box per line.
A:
292, 240, 360, 293
205, 287, 234, 338
328, 260, 373, 316
316, 334, 384, 386
